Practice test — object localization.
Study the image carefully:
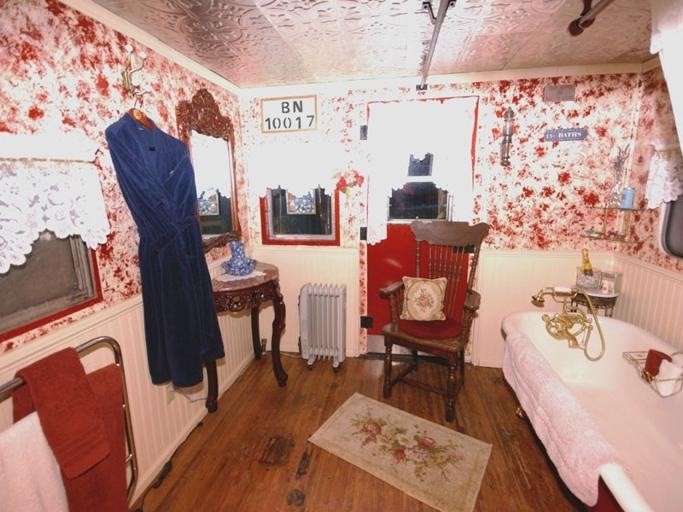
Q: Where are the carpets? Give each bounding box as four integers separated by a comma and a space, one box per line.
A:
307, 391, 494, 512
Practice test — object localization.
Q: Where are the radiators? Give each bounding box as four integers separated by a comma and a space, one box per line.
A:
296, 283, 347, 371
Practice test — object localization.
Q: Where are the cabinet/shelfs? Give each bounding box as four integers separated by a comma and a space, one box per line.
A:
589, 205, 644, 243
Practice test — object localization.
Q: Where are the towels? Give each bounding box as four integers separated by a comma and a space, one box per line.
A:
1, 347, 134, 512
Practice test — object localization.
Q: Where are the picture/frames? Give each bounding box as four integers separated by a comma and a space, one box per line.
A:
260, 186, 341, 246
260, 94, 318, 133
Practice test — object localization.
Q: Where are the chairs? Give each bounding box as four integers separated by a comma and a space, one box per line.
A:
380, 219, 489, 422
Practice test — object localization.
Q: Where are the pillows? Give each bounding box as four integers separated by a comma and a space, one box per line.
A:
400, 275, 448, 322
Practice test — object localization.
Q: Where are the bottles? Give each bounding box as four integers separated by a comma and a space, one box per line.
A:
619, 186, 636, 209
582, 248, 593, 277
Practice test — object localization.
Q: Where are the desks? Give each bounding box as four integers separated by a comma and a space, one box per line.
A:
204, 260, 287, 414
571, 289, 617, 318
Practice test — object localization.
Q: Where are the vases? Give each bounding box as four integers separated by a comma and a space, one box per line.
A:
222, 241, 256, 275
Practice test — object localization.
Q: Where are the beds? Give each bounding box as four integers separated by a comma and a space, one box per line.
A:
499, 309, 683, 512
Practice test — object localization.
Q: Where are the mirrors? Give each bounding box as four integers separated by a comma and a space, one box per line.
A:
175, 88, 243, 253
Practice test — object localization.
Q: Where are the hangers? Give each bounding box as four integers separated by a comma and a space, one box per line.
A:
127, 92, 154, 131
